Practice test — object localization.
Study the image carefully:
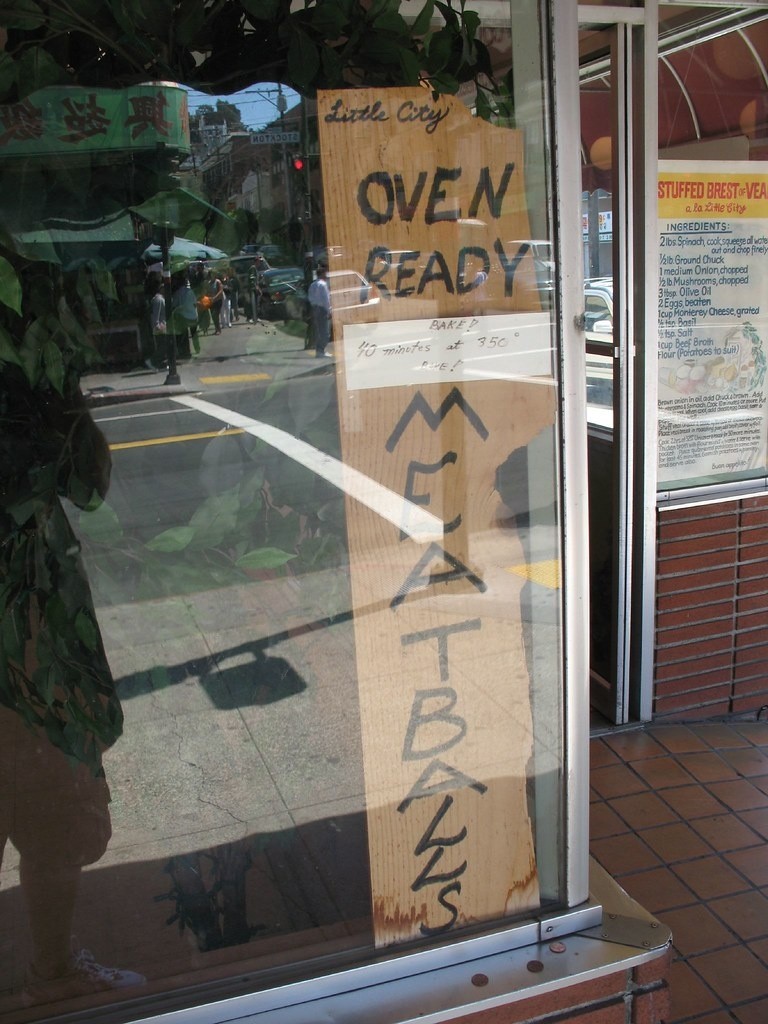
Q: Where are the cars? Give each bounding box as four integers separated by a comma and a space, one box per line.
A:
243, 267, 306, 317
584, 277, 615, 383
283, 271, 382, 336
192, 259, 219, 277
499, 240, 554, 293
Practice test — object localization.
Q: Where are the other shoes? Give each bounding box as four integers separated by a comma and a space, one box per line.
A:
222, 325, 225, 329
316, 353, 331, 359
213, 330, 220, 335
226, 322, 232, 328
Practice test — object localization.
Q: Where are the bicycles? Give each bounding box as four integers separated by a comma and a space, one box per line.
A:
194, 301, 209, 336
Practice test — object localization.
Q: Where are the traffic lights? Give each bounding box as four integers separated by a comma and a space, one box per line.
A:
292, 153, 306, 187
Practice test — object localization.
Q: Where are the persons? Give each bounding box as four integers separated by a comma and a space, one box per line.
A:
146, 260, 262, 368
0, 244, 148, 1006
308, 267, 335, 358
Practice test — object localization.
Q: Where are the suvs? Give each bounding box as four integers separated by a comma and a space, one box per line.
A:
224, 256, 272, 299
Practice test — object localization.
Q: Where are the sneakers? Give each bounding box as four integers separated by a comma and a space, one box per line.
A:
19, 935, 147, 1008
